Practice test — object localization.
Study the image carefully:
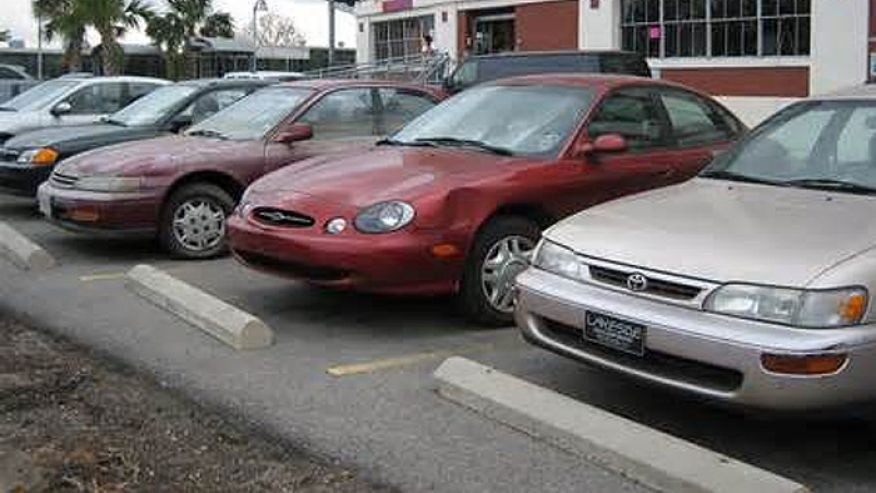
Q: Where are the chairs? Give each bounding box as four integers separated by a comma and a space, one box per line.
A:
294, 96, 378, 144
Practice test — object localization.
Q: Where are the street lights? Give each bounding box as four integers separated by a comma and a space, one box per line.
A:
253, 1, 267, 73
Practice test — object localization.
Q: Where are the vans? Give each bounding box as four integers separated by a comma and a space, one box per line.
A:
442, 51, 651, 99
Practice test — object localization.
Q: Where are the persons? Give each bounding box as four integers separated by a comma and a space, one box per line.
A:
420, 31, 433, 58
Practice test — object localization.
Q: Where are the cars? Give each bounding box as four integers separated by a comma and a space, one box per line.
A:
0, 62, 874, 409
